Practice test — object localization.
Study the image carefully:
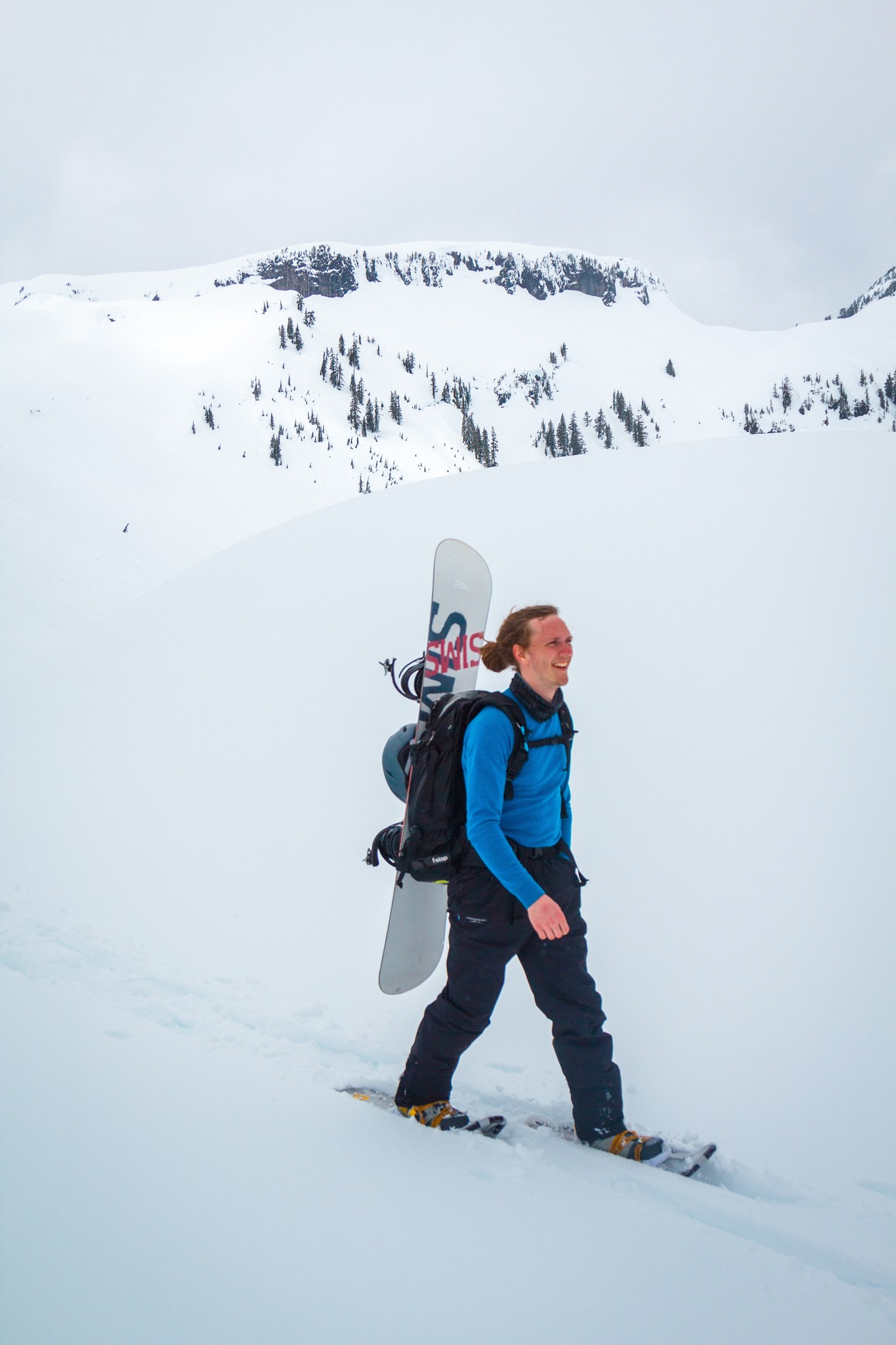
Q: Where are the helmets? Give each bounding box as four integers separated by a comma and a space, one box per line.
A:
383, 724, 417, 804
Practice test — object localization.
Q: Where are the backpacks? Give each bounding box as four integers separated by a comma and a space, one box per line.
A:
399, 690, 572, 880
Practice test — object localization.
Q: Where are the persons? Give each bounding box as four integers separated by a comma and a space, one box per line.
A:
394, 605, 673, 1168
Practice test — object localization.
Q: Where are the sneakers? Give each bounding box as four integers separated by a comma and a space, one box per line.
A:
583, 1128, 661, 1163
399, 1099, 469, 1131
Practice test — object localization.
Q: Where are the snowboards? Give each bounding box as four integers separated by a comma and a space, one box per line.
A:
377, 541, 495, 997
